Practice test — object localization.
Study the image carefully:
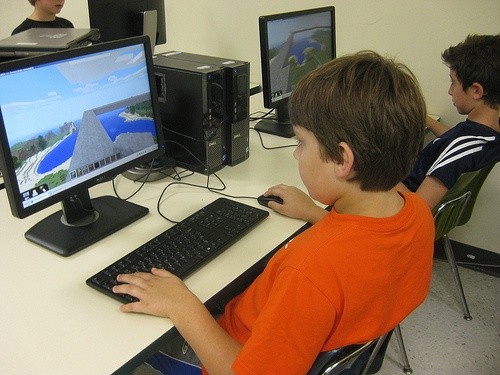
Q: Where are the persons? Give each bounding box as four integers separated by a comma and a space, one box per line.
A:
11, 0, 74, 36
113, 49, 435, 375
396, 32, 500, 208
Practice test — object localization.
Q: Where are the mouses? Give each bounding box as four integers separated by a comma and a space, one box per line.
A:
257, 195, 282, 208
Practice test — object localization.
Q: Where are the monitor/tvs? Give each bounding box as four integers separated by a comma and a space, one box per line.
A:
0, 35, 165, 257
88, 0, 167, 57
255, 6, 337, 137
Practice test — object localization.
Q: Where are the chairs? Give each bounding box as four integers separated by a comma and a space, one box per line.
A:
430, 161, 498, 321
306, 321, 413, 375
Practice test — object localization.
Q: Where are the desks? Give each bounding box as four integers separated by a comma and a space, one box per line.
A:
0, 90, 440, 375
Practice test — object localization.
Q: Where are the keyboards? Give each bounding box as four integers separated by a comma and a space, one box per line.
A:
86, 197, 269, 304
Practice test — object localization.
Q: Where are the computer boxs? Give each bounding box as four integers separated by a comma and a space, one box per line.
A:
152, 57, 230, 174
154, 51, 250, 166
